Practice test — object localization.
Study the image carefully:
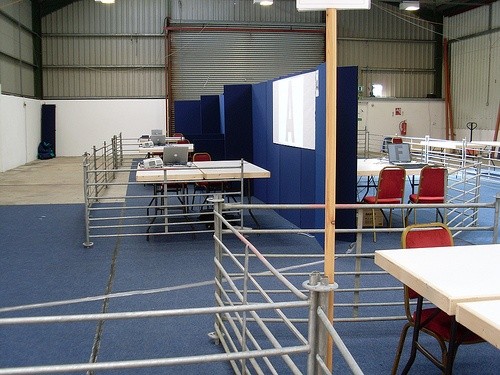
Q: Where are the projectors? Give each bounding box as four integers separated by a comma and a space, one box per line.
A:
142, 135, 149, 139
142, 141, 154, 147
144, 157, 163, 167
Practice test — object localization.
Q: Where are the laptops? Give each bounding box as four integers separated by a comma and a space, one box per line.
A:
162, 146, 189, 165
386, 143, 411, 164
150, 136, 166, 146
152, 130, 162, 135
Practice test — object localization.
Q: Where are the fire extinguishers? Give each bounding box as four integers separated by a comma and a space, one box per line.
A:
399, 119, 407, 137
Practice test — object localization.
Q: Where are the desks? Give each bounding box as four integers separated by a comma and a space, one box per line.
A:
137, 143, 194, 187
471, 141, 500, 171
357, 159, 447, 233
455, 299, 500, 351
374, 244, 500, 375
136, 159, 271, 241
420, 140, 485, 178
137, 137, 185, 159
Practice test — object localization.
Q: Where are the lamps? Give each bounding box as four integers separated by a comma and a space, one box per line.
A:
399, 1, 420, 11
253, 0, 273, 6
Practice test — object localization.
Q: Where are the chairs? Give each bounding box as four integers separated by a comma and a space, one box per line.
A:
364, 166, 406, 243
406, 164, 448, 225
390, 222, 487, 375
154, 132, 212, 211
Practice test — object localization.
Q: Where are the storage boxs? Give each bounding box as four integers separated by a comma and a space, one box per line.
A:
363, 208, 384, 227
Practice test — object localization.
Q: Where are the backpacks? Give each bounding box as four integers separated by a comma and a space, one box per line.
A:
38, 141, 54, 159
